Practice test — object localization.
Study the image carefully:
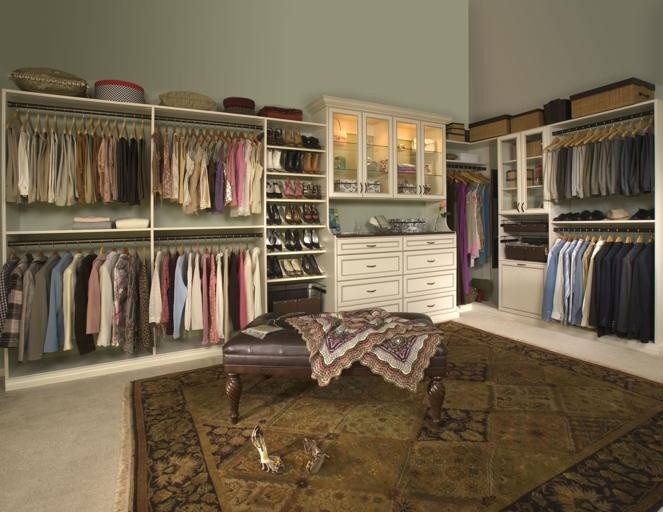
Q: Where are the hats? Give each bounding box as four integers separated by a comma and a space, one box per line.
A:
605, 208, 631, 220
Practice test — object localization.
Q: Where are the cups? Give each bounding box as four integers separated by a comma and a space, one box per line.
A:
423, 164, 432, 175
334, 157, 345, 169
533, 162, 542, 186
366, 161, 376, 172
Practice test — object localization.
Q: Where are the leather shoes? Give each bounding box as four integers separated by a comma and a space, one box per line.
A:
554, 211, 602, 221
631, 209, 654, 220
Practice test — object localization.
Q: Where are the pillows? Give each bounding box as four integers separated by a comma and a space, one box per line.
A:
95, 79, 145, 103
8, 65, 87, 97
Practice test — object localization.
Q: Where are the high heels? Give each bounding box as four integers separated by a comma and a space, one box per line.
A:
267, 177, 322, 198
304, 436, 330, 474
266, 229, 320, 251
267, 255, 321, 279
267, 126, 321, 149
251, 425, 285, 474
267, 203, 320, 224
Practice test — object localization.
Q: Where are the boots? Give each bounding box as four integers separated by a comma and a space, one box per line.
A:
267, 149, 321, 174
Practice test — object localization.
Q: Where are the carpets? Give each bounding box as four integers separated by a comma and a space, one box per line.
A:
116, 320, 663, 512
